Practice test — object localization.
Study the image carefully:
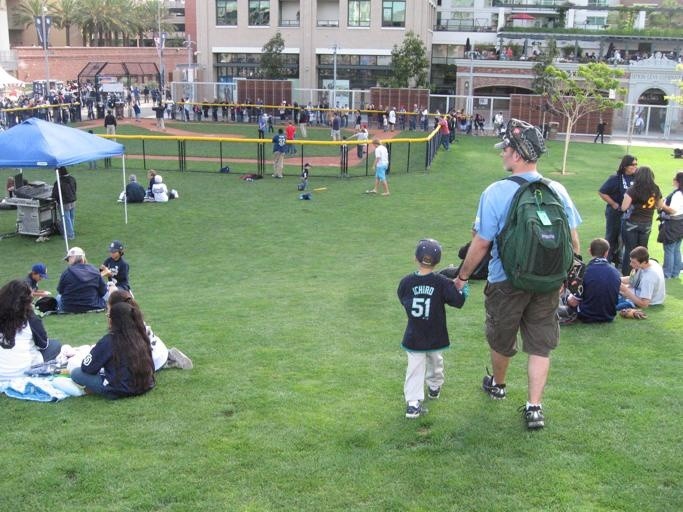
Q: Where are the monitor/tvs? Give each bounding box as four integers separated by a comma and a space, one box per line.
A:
14, 173, 24, 190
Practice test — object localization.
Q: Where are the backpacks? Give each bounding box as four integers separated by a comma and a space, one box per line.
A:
493, 176, 573, 292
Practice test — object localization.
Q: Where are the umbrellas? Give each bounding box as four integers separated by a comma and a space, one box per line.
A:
509, 11, 536, 27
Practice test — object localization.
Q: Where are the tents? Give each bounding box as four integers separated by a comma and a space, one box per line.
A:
0, 115, 127, 247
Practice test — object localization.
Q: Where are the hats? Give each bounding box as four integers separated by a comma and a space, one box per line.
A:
155, 175, 162, 183
495, 118, 547, 162
416, 237, 442, 266
32, 263, 49, 279
107, 241, 123, 252
62, 247, 84, 260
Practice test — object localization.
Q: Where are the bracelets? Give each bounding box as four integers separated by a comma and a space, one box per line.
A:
457, 273, 469, 282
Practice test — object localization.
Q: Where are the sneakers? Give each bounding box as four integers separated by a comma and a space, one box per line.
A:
483, 375, 506, 400
406, 406, 429, 418
169, 346, 193, 370
523, 402, 545, 429
428, 386, 440, 398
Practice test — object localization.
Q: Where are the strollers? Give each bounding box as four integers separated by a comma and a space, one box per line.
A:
498, 124, 506, 139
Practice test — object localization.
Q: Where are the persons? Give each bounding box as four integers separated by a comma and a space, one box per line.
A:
104, 108, 116, 142
372, 138, 390, 196
126, 168, 178, 202
70, 302, 156, 401
453, 45, 683, 66
564, 238, 622, 325
294, 100, 405, 157
450, 117, 581, 432
100, 241, 134, 295
615, 245, 667, 309
80, 82, 125, 120
193, 97, 263, 124
634, 114, 645, 136
126, 86, 190, 129
109, 291, 194, 372
0, 279, 62, 378
51, 165, 78, 240
271, 128, 287, 177
619, 165, 664, 277
23, 262, 51, 299
396, 237, 470, 422
593, 118, 607, 144
258, 103, 292, 139
54, 247, 109, 314
656, 172, 683, 277
413, 104, 502, 150
284, 121, 296, 156
439, 222, 498, 280
596, 153, 639, 269
1, 82, 81, 128
302, 163, 311, 190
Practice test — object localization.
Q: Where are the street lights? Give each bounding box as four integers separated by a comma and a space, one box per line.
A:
465, 51, 481, 124
176, 34, 196, 67
328, 42, 343, 108
36, 5, 51, 96
